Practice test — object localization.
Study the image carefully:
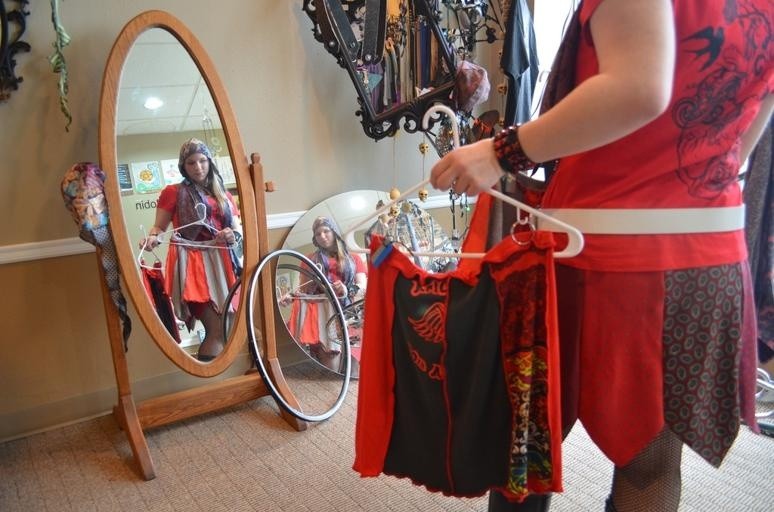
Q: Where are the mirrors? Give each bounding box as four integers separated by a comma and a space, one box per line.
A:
97, 9, 304, 480
271, 188, 458, 384
303, 0, 467, 137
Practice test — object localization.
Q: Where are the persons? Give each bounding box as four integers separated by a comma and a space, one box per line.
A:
430, 0, 774, 510
139, 138, 242, 364
279, 217, 368, 378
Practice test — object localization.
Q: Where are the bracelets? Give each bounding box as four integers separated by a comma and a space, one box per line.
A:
493, 123, 535, 173
289, 292, 294, 302
149, 234, 158, 236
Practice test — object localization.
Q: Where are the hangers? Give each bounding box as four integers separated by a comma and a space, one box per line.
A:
345, 98, 588, 263
278, 262, 349, 302
131, 221, 163, 272
156, 201, 241, 250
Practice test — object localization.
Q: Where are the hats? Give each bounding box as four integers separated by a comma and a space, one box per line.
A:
178, 139, 224, 230
434, 61, 501, 153
312, 216, 350, 253
